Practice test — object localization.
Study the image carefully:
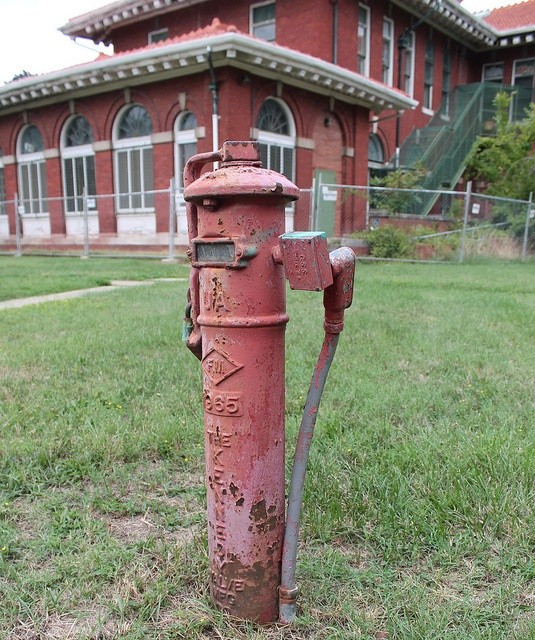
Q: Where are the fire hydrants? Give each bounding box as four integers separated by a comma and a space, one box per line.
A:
176, 138, 357, 630
